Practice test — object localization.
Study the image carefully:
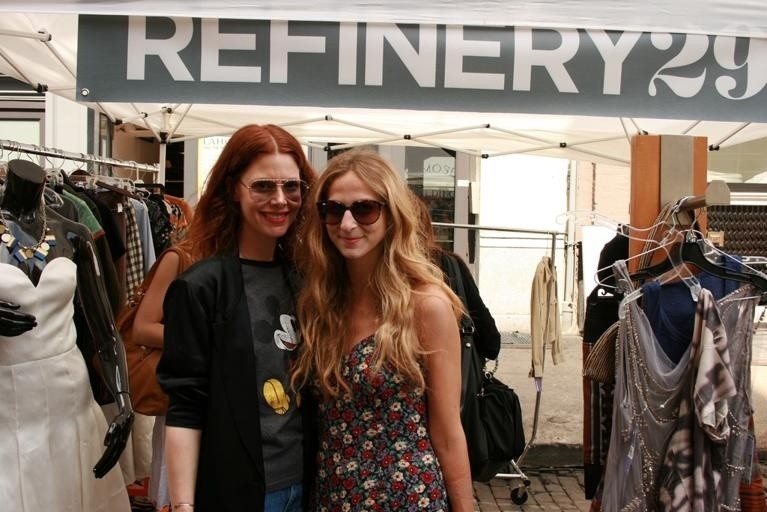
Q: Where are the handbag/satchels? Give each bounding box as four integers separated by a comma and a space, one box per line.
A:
460, 319, 525, 483
115, 246, 185, 415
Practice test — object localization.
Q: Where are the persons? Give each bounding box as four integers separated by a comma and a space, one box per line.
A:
0, 160, 134, 512
289, 151, 478, 512
419, 198, 500, 360
156, 125, 317, 512
130, 240, 202, 512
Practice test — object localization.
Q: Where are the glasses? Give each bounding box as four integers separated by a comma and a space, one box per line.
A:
316, 199, 387, 224
238, 177, 310, 202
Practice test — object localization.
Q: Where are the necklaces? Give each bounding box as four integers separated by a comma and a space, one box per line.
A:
0, 207, 56, 263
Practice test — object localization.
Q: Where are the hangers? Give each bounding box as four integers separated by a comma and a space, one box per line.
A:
0, 136, 168, 210
571, 192, 767, 320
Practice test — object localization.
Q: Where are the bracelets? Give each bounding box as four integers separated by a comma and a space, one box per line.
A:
172, 503, 196, 509
